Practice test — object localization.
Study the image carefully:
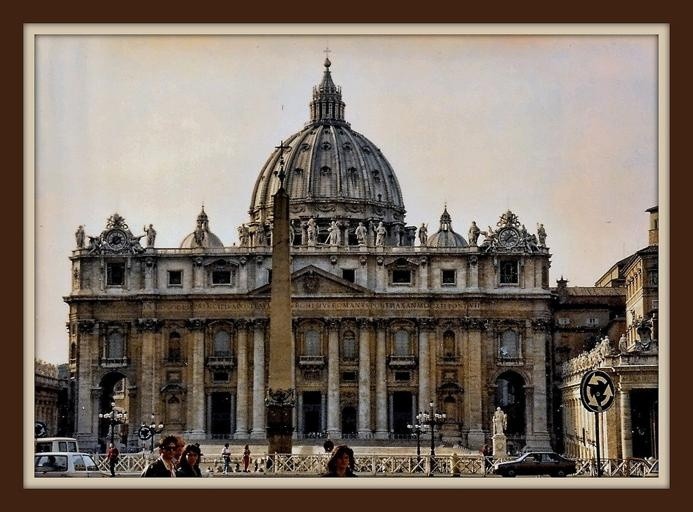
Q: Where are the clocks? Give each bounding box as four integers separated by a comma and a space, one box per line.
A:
107, 232, 125, 249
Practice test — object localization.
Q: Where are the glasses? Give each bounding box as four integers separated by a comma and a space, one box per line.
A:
162, 445, 176, 451
186, 453, 199, 458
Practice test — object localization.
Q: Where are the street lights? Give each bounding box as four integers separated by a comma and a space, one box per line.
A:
99, 403, 130, 444
142, 414, 164, 452
408, 403, 448, 476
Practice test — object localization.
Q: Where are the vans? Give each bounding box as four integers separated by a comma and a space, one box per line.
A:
34, 437, 80, 453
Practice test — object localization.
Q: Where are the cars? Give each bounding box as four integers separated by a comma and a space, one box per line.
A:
492, 452, 577, 477
34, 453, 109, 477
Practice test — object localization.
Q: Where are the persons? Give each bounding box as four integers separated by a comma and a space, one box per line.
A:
468, 220, 480, 245
496, 407, 509, 434
373, 223, 387, 246
139, 434, 203, 478
306, 218, 319, 241
377, 228, 385, 245
308, 428, 329, 440
238, 222, 272, 245
144, 224, 155, 247
418, 223, 428, 244
319, 445, 358, 477
560, 334, 627, 378
76, 225, 85, 250
354, 222, 368, 246
222, 443, 231, 471
520, 223, 548, 254
327, 221, 343, 244
243, 444, 251, 472
105, 442, 118, 477
